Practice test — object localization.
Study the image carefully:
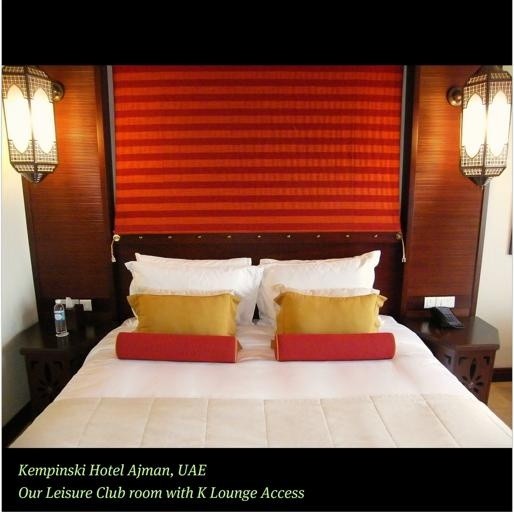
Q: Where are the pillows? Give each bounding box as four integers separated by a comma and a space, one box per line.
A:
115, 251, 396, 363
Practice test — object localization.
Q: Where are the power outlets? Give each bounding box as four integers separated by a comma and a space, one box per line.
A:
54, 298, 93, 311
423, 295, 457, 309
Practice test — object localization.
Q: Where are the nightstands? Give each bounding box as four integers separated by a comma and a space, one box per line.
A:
400, 315, 500, 407
18, 322, 114, 421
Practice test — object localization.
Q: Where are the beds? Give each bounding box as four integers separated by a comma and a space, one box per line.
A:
7, 230, 513, 450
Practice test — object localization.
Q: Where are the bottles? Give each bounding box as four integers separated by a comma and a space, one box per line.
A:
53, 299, 68, 337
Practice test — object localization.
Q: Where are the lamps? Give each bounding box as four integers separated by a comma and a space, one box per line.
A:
1, 64, 65, 187
445, 65, 512, 190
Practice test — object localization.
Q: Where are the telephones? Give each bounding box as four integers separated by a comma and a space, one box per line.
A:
430, 307, 464, 328
